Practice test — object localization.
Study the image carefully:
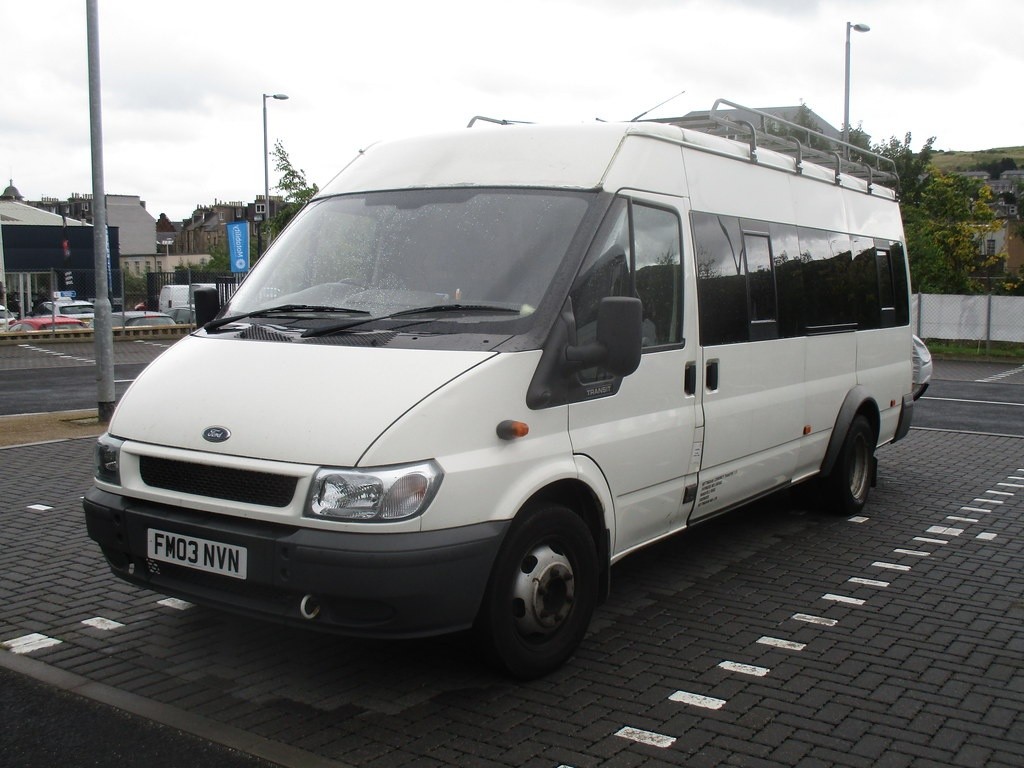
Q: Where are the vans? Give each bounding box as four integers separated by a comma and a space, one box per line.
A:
159, 283, 243, 324
80, 100, 914, 682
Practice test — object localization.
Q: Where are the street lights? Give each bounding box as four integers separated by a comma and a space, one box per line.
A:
263, 94, 290, 254
162, 237, 174, 272
841, 21, 870, 160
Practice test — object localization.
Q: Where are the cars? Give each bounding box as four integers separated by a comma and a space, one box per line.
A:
0, 305, 17, 328
162, 304, 196, 324
1, 317, 91, 339
87, 311, 178, 336
25, 300, 94, 319
